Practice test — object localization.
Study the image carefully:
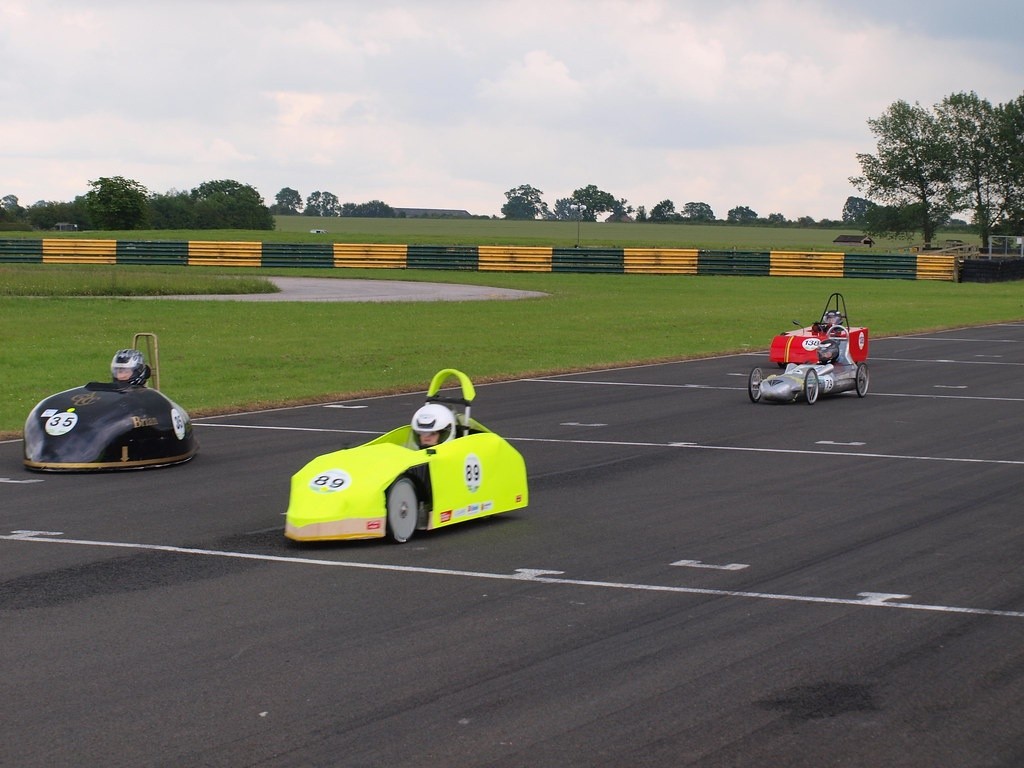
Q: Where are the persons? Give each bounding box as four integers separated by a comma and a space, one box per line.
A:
409, 401, 458, 458
809, 339, 845, 383
109, 347, 146, 390
823, 308, 845, 337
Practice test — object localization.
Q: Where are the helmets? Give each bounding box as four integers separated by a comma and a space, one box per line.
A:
824, 310, 842, 325
111, 349, 152, 387
411, 404, 457, 451
816, 339, 840, 363
